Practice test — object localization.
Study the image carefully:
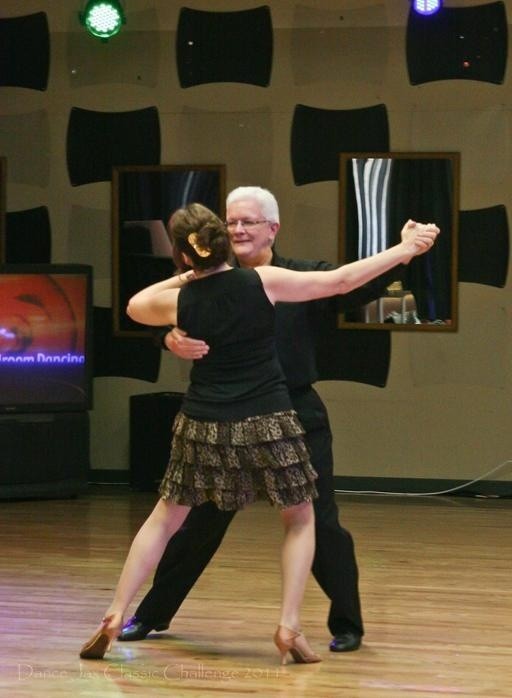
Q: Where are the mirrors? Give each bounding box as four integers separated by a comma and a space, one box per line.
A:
337, 152, 460, 332
112, 163, 226, 337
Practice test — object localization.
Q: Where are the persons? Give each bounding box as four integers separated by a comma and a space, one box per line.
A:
79, 201, 437, 667
116, 185, 440, 652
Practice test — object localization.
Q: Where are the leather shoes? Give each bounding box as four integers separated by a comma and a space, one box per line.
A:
117, 614, 169, 641
329, 630, 361, 652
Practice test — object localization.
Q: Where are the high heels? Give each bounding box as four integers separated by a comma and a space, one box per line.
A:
275, 624, 324, 665
80, 613, 123, 659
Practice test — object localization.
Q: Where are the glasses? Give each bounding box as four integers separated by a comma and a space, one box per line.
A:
223, 219, 272, 229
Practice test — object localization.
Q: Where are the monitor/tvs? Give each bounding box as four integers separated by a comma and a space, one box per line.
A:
0, 263, 94, 414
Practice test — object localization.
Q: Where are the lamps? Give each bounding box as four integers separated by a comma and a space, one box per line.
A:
411, 0, 442, 16
79, 0, 125, 42
